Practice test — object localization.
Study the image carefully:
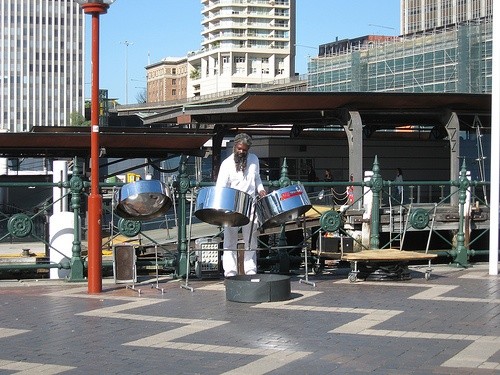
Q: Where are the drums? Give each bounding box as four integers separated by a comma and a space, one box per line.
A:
112, 178, 174, 224
193, 188, 253, 228
256, 180, 312, 227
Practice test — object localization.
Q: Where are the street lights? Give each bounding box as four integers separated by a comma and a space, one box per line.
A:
72, 0, 117, 295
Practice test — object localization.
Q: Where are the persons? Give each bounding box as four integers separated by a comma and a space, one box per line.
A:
305, 162, 319, 193
393, 168, 404, 204
214, 133, 267, 282
